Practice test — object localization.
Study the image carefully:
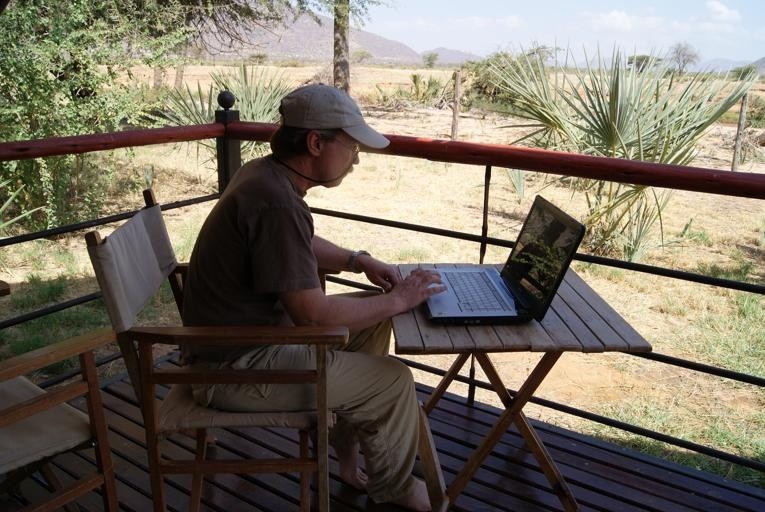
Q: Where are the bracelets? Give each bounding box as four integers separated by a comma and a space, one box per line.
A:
349, 250, 370, 272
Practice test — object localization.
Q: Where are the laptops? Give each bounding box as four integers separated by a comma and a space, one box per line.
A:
423, 194, 586, 327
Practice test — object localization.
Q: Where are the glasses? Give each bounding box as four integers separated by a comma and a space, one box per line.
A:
318, 131, 360, 156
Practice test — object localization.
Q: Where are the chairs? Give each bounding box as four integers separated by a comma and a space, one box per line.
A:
85, 188, 350, 510
1, 280, 119, 510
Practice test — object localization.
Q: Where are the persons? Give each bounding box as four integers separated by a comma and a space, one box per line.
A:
182, 83, 447, 512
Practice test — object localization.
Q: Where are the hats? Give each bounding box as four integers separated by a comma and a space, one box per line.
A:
280, 82, 391, 149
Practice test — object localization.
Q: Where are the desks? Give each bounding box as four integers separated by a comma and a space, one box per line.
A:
375, 255, 651, 512
